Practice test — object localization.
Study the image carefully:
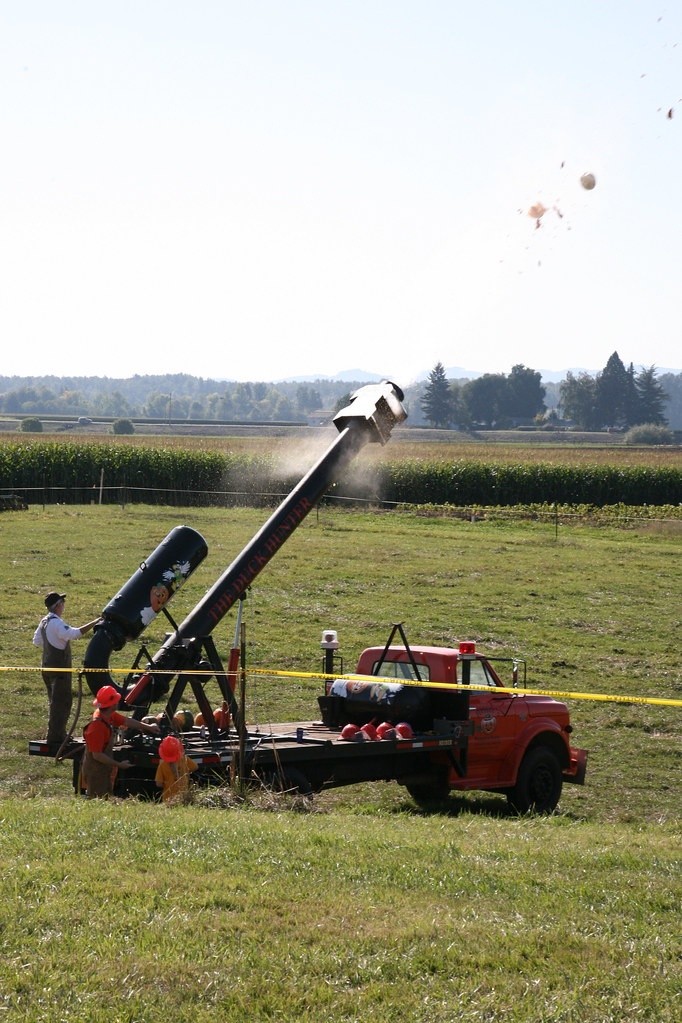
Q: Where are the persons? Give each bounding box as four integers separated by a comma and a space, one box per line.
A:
154, 735, 198, 806
32, 592, 103, 745
78, 686, 160, 798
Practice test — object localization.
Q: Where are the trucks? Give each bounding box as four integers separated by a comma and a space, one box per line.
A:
29, 376, 589, 818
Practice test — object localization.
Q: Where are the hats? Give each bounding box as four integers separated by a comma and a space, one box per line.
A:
45, 592, 66, 607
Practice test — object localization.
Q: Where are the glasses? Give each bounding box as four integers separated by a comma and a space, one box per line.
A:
56, 599, 65, 608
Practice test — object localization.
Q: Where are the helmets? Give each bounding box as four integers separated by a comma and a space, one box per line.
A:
92, 685, 121, 708
158, 735, 183, 763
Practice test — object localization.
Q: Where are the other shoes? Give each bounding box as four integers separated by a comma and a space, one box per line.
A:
64, 734, 77, 742
46, 739, 70, 747
